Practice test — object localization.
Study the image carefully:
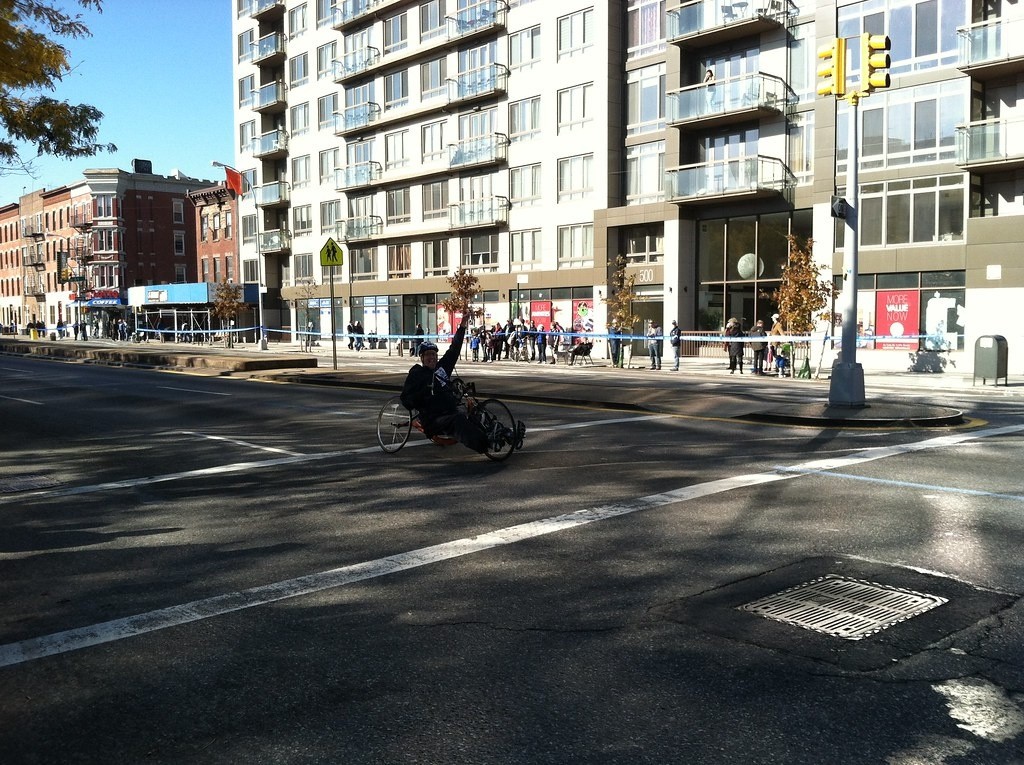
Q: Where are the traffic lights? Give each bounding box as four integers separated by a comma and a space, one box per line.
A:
861, 33, 891, 93
816, 38, 846, 96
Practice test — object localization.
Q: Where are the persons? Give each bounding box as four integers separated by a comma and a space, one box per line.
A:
413, 323, 424, 357
400, 312, 525, 454
608, 318, 626, 368
703, 70, 717, 113
347, 319, 365, 351
471, 317, 591, 366
156, 318, 165, 342
647, 320, 663, 370
180, 321, 191, 343
104, 318, 132, 341
670, 319, 682, 371
80, 321, 88, 341
57, 319, 64, 339
74, 321, 79, 341
94, 323, 100, 339
25, 320, 46, 337
724, 313, 794, 379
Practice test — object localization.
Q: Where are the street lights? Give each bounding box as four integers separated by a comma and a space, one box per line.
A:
211, 160, 267, 351
45, 233, 82, 341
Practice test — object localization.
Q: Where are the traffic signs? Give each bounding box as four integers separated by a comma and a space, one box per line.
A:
831, 196, 847, 219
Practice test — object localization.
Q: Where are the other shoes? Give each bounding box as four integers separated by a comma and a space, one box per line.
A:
740, 371, 743, 374
544, 361, 547, 364
730, 370, 734, 374
669, 367, 678, 371
513, 420, 525, 450
656, 368, 661, 370
549, 361, 555, 364
537, 361, 542, 364
568, 363, 573, 365
726, 367, 732, 370
752, 368, 758, 374
758, 370, 766, 375
769, 368, 771, 371
650, 367, 656, 370
490, 422, 504, 452
764, 368, 767, 371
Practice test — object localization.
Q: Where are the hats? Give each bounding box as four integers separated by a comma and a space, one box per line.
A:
771, 313, 779, 318
728, 318, 737, 322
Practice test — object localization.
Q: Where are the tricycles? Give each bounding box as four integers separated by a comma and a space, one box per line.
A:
375, 377, 527, 462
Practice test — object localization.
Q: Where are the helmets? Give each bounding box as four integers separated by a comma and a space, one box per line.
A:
419, 341, 438, 355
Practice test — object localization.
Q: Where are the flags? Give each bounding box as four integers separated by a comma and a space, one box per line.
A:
223, 167, 244, 195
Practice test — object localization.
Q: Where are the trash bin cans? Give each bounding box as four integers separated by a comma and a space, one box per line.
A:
30, 329, 38, 340
973, 335, 1008, 387
50, 333, 56, 341
